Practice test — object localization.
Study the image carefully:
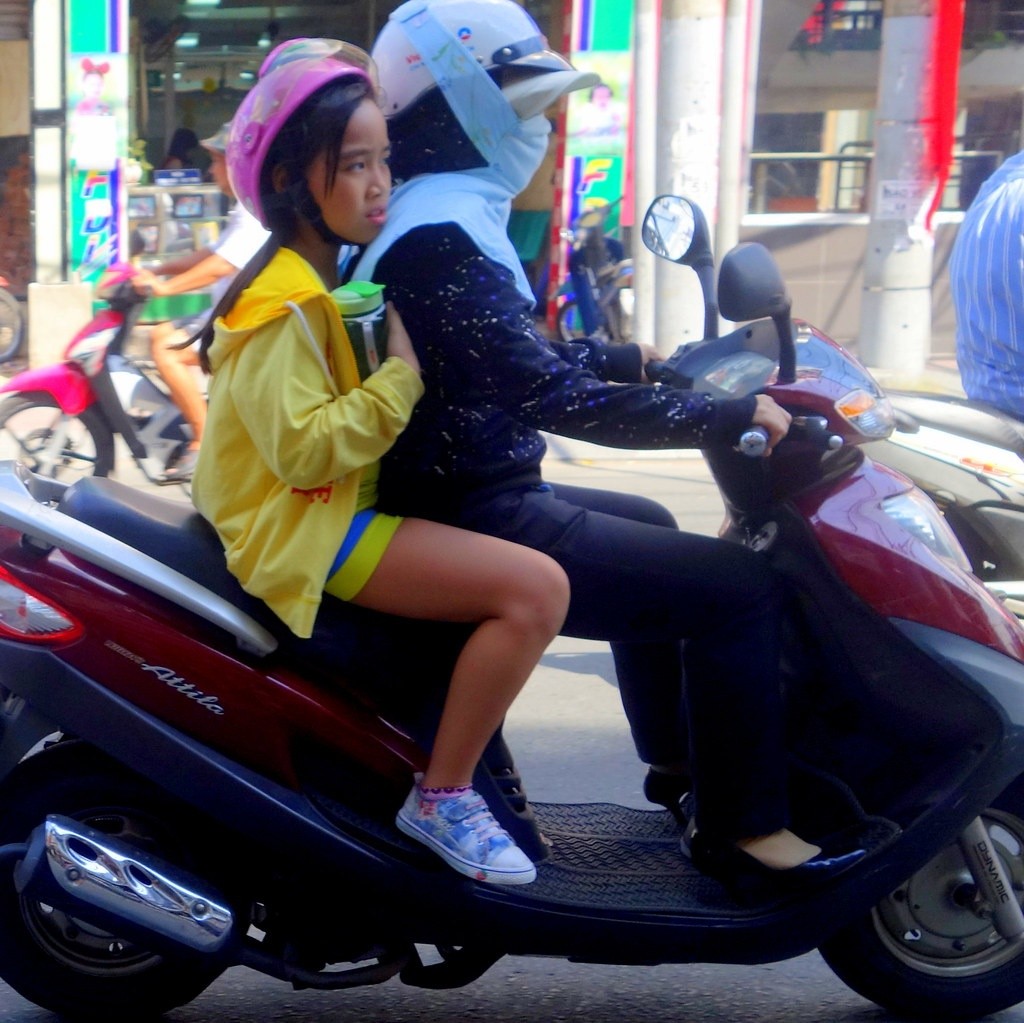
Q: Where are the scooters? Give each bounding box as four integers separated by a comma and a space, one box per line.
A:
1, 189, 1024, 1023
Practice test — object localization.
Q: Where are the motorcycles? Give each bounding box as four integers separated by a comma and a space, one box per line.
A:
0, 229, 217, 508
845, 384, 1024, 624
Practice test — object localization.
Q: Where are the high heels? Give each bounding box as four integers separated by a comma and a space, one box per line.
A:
682, 817, 903, 901
642, 766, 705, 824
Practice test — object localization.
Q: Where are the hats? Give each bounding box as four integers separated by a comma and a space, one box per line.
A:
198, 122, 230, 156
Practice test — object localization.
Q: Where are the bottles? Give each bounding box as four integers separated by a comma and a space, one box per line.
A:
331, 278, 386, 377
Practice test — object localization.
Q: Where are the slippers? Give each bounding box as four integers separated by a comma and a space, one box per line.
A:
164, 443, 202, 480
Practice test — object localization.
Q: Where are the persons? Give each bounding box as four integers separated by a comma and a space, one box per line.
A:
336, 0, 871, 907
948, 149, 1024, 423
224, 37, 570, 885
129, 121, 272, 479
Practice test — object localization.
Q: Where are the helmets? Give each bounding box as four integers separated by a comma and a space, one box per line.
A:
226, 56, 373, 231
368, 0, 550, 120
257, 40, 327, 76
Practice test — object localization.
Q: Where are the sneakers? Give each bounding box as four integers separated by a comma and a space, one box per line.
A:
395, 772, 537, 884
